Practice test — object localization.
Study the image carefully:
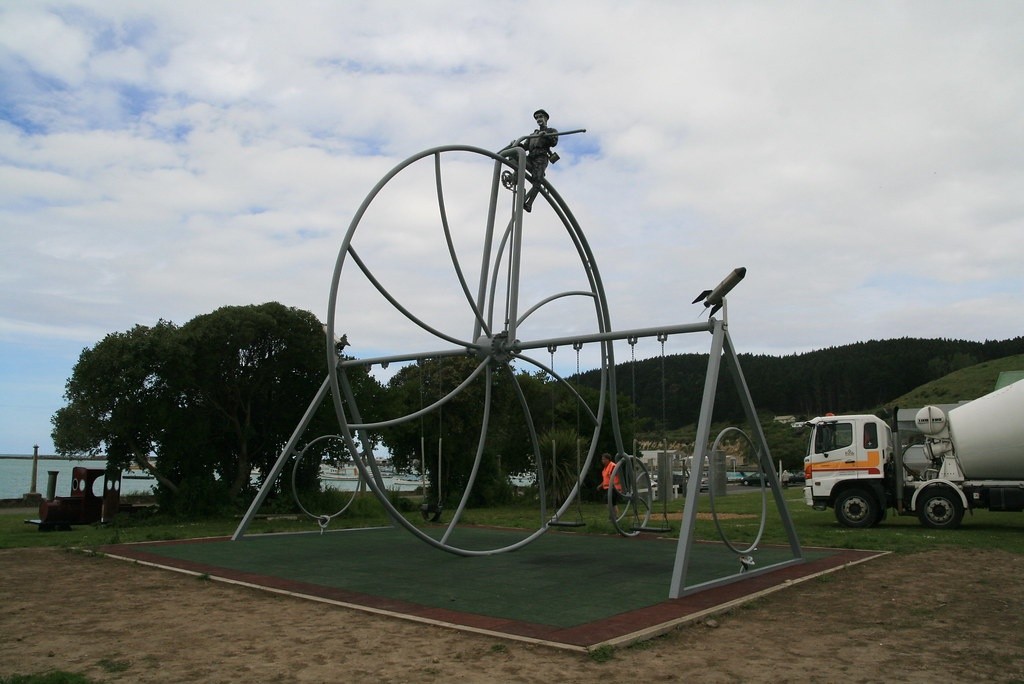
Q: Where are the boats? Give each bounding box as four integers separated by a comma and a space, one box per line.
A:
320, 463, 430, 484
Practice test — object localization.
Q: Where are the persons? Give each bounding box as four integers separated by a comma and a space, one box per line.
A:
510, 109, 560, 212
782, 471, 789, 488
596, 454, 623, 518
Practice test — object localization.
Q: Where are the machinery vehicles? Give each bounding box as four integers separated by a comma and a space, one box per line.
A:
799, 377, 1023, 528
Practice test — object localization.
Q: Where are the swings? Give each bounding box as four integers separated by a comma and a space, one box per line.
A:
548, 350, 587, 528
630, 340, 672, 533
418, 358, 443, 523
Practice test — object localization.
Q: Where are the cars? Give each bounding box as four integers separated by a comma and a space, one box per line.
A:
636, 458, 806, 499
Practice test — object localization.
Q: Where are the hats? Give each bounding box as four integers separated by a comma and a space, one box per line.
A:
533, 109, 549, 120
